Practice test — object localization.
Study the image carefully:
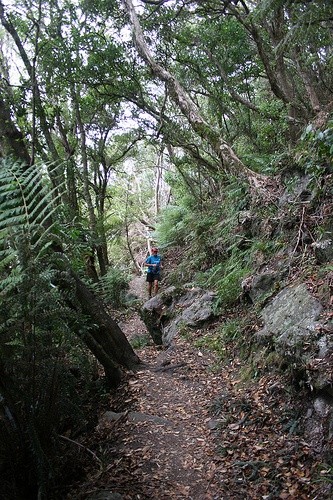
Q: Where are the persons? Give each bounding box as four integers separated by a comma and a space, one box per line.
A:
143, 247, 166, 300
